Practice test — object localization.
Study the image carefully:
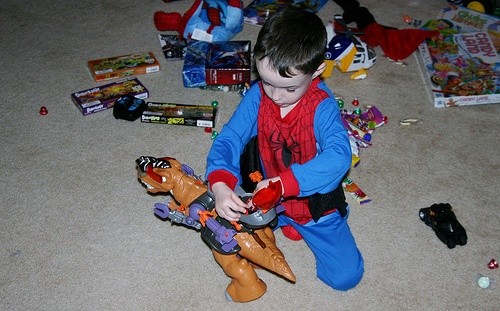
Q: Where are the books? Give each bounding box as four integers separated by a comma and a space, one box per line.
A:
88, 51, 160, 83
71, 76, 149, 116
182, 42, 250, 88
141, 102, 217, 128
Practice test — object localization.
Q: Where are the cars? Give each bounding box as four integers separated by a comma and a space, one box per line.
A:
419, 203, 468, 249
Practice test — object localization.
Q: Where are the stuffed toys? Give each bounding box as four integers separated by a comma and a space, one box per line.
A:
333, 6, 438, 63
325, 23, 376, 79
154, 0, 246, 44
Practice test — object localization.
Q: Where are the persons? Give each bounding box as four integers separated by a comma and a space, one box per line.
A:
205, 5, 364, 290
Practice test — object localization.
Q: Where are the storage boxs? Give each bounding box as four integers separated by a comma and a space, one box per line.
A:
85, 51, 160, 80
184, 40, 253, 86
71, 77, 148, 117
141, 101, 220, 128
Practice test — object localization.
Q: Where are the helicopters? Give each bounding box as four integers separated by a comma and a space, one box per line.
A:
321, 28, 377, 81
